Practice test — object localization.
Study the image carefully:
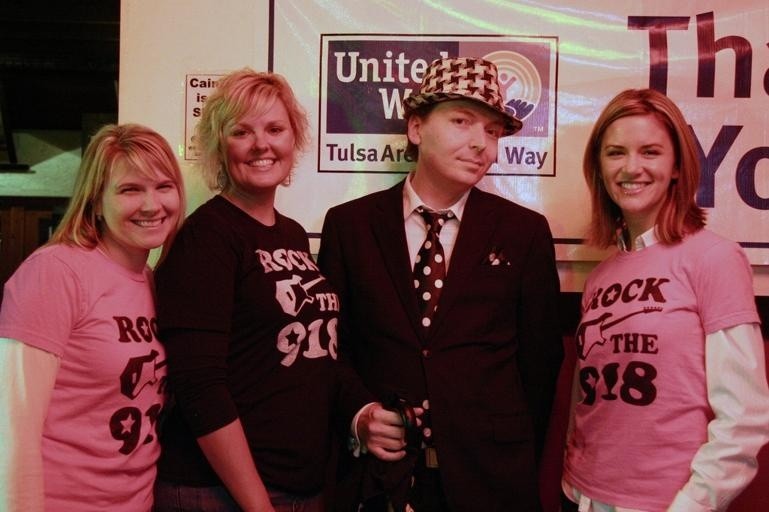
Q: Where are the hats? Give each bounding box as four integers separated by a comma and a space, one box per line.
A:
398, 56, 523, 141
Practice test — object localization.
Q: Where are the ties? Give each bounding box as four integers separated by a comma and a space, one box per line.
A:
400, 207, 459, 450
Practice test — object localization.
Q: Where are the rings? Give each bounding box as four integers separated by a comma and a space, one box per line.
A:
368, 405, 383, 417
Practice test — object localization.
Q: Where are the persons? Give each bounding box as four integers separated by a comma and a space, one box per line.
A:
539, 85, 768, 511
0, 121, 188, 510
316, 53, 569, 511
152, 66, 362, 510
